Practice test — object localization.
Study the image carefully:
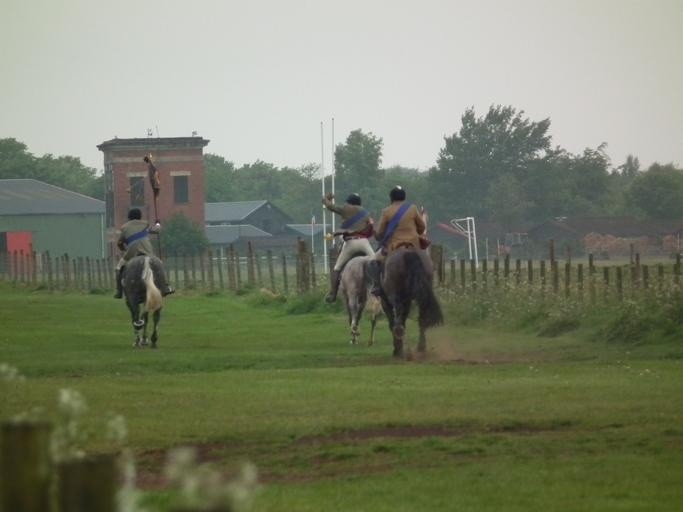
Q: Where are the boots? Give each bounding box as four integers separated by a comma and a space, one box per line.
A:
164, 285, 175, 294
326, 271, 340, 303
113, 269, 123, 298
370, 267, 381, 294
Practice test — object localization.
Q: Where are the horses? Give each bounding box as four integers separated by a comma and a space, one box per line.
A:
378, 247, 444, 358
337, 234, 385, 348
121, 254, 165, 348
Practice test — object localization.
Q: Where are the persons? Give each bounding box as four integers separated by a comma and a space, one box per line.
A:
320, 192, 377, 303
369, 185, 426, 293
113, 208, 175, 299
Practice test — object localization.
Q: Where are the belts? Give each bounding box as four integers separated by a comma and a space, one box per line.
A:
344, 233, 368, 239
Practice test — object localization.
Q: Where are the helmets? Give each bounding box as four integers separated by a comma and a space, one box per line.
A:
345, 192, 361, 205
389, 186, 406, 201
127, 207, 142, 219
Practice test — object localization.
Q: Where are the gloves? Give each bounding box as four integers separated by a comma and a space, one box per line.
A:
154, 220, 160, 229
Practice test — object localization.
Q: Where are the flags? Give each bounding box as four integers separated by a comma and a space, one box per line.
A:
141, 157, 161, 196
